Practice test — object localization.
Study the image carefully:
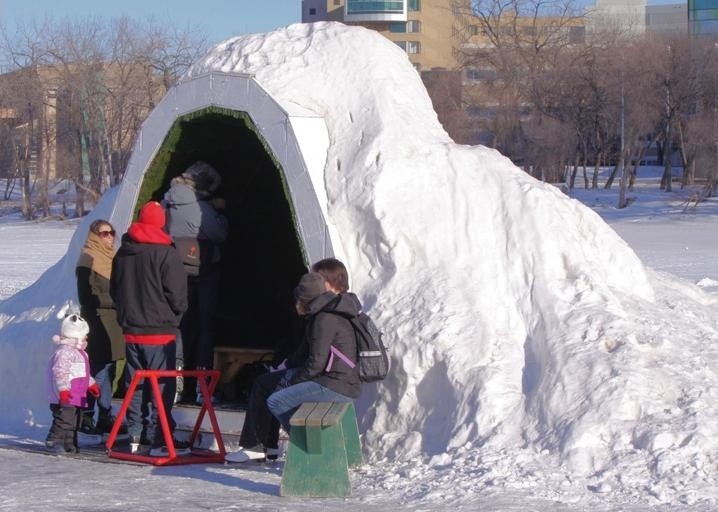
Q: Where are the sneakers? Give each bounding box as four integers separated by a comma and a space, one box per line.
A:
149, 440, 192, 458
129, 435, 156, 457
264, 447, 279, 464
223, 443, 266, 467
195, 392, 220, 406
173, 392, 194, 405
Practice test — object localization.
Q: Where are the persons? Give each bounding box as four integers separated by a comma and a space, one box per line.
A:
110, 200, 189, 449
78, 219, 126, 434
45, 313, 101, 455
267, 273, 361, 437
158, 160, 229, 404
223, 258, 362, 464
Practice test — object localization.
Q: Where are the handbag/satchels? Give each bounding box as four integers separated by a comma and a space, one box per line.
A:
237, 353, 284, 405
174, 236, 215, 279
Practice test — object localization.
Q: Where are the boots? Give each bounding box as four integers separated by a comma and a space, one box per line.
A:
43, 433, 65, 456
96, 406, 129, 434
64, 433, 81, 454
77, 410, 103, 436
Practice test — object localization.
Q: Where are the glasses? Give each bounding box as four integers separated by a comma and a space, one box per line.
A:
98, 229, 115, 238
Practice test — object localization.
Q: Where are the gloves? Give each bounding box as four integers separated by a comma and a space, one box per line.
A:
58, 390, 74, 405
87, 383, 100, 398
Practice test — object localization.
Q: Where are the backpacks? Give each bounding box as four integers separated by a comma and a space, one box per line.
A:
348, 312, 391, 383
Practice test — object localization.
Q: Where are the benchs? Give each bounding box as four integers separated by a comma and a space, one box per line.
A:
278, 401, 365, 497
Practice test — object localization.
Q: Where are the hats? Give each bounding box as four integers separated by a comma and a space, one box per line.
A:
137, 201, 166, 229
182, 160, 222, 193
60, 313, 90, 341
292, 272, 327, 302
